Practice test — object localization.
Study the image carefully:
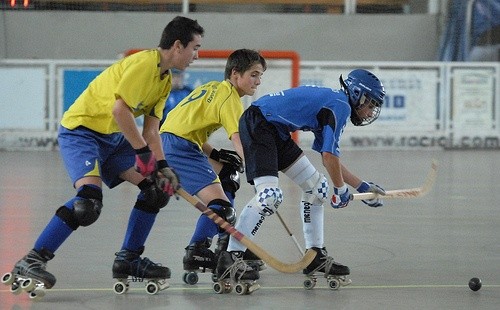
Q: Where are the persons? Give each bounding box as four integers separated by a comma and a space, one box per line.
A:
159, 48, 267, 285
0, 16, 206, 301
159, 67, 195, 132
211, 68, 386, 295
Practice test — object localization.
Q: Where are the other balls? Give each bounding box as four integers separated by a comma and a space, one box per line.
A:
467, 277, 483, 291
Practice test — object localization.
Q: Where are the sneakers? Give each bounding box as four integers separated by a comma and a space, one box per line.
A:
183, 238, 215, 285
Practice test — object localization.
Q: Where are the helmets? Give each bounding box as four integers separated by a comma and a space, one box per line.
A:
340, 69, 385, 108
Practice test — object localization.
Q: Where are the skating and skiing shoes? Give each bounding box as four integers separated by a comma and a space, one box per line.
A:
1, 247, 56, 303
112, 246, 172, 295
213, 251, 261, 295
215, 238, 266, 271
304, 247, 352, 290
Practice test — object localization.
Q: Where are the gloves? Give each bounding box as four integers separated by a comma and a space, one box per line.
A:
210, 148, 244, 173
152, 160, 180, 196
355, 180, 386, 207
133, 144, 158, 177
331, 187, 349, 208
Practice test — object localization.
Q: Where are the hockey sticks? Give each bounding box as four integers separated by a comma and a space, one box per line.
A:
348, 159, 439, 202
156, 171, 319, 276
252, 184, 305, 258
